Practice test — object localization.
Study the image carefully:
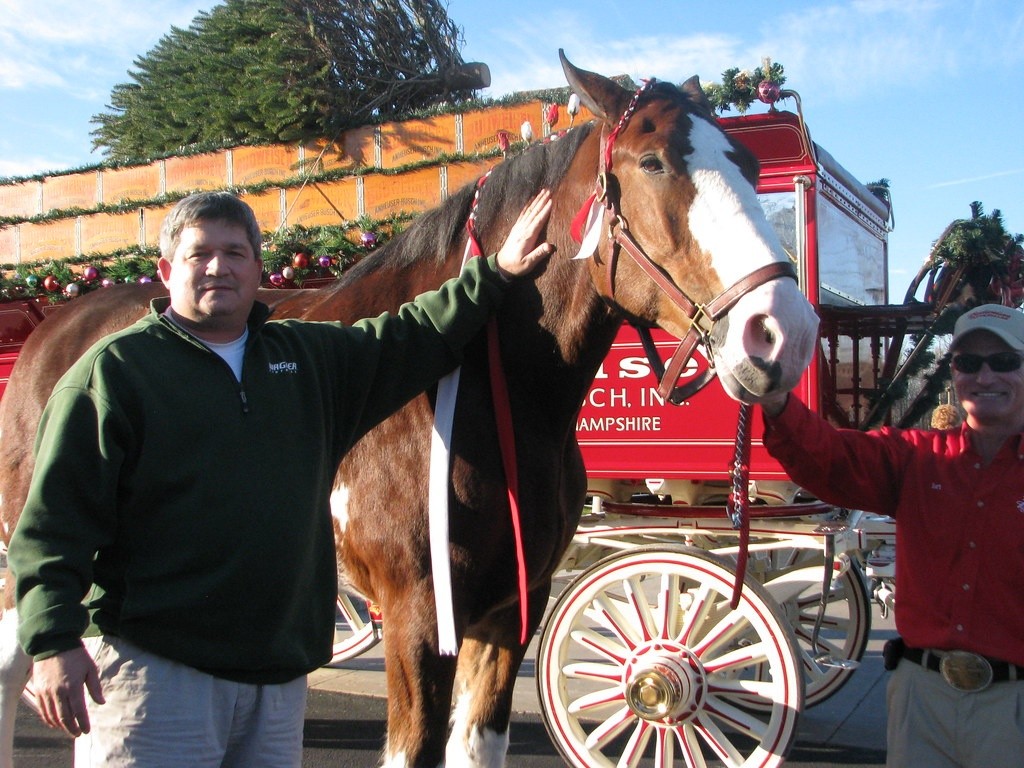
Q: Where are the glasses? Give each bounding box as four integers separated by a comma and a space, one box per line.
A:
948, 353, 1023, 373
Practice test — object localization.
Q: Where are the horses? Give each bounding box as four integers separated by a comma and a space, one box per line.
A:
0, 47, 821, 768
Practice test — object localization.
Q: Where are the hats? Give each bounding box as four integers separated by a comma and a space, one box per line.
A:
949, 303, 1023, 355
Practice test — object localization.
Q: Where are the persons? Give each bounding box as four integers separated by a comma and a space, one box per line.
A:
760, 303, 1024, 768
15, 188, 554, 766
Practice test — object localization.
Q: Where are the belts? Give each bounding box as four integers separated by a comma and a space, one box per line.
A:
904, 644, 1023, 693
